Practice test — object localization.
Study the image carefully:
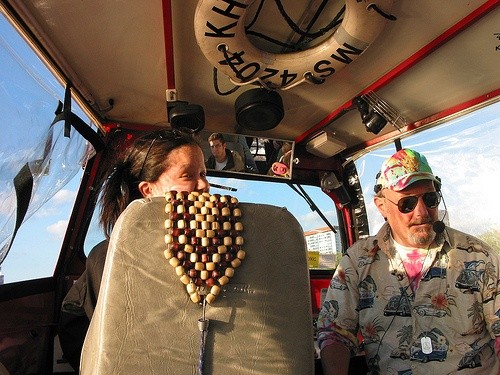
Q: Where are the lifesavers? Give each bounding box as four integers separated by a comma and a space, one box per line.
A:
193, 0, 391, 92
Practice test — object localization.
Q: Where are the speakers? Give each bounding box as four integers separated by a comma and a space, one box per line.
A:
235, 88, 285, 131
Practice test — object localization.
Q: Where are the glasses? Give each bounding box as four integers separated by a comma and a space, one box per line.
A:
139, 128, 189, 180
381, 192, 441, 213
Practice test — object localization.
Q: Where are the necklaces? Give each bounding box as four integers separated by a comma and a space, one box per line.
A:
402, 251, 443, 354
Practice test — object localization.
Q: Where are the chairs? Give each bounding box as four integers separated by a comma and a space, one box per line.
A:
79, 197, 315, 375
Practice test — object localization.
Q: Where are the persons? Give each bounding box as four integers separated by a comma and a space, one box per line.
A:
317, 149, 500, 375
204, 133, 259, 174
57, 131, 209, 374
262, 139, 292, 178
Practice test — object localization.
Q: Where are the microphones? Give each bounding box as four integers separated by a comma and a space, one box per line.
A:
433, 190, 447, 233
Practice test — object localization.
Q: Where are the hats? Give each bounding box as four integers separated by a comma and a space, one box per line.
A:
379, 149, 440, 191
